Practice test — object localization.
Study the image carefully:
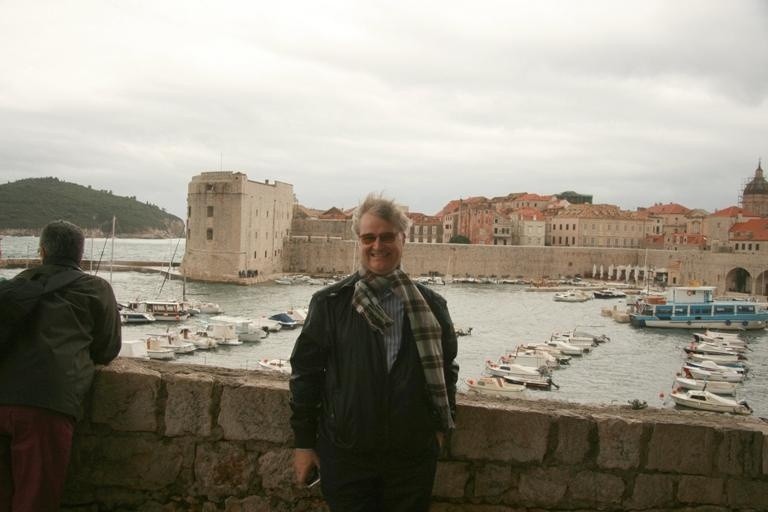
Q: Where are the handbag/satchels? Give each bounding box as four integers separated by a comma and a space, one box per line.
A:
0, 268, 84, 364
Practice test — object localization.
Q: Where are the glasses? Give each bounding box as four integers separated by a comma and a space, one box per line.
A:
359, 232, 398, 244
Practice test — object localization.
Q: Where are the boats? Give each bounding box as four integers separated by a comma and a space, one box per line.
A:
667, 330, 755, 417
552, 286, 627, 302
459, 326, 608, 396
275, 272, 347, 287
117, 298, 308, 375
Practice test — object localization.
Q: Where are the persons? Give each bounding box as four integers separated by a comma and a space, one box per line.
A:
1, 219, 122, 512
290, 192, 460, 512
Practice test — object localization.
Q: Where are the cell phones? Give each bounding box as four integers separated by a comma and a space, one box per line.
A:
305, 466, 321, 490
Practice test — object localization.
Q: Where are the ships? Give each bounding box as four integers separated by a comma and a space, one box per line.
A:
628, 301, 768, 331
